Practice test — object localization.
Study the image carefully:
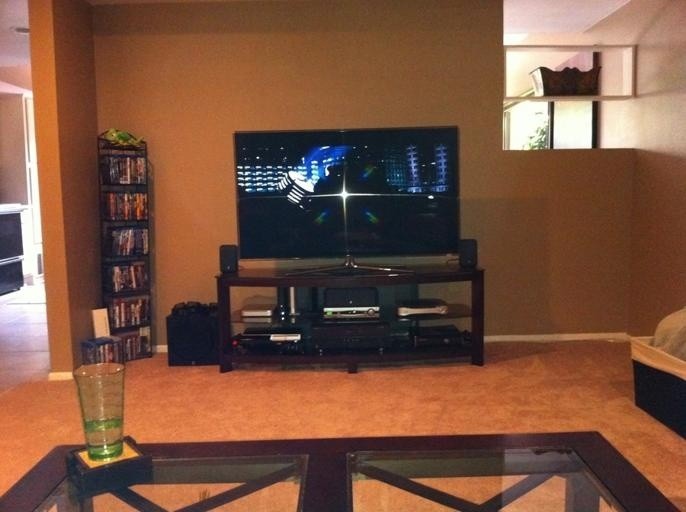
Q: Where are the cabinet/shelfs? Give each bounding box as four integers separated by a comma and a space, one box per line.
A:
95, 137, 152, 364
214, 264, 486, 371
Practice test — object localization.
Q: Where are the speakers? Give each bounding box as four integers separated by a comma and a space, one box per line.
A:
457, 239, 476, 269
165, 305, 220, 366
219, 245, 237, 273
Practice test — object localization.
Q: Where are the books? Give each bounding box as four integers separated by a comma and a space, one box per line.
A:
102, 153, 152, 360
82, 336, 121, 367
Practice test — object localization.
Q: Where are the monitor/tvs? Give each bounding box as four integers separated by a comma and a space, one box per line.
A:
233, 125, 460, 279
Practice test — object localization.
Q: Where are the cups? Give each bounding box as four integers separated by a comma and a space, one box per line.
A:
73, 361, 125, 462
